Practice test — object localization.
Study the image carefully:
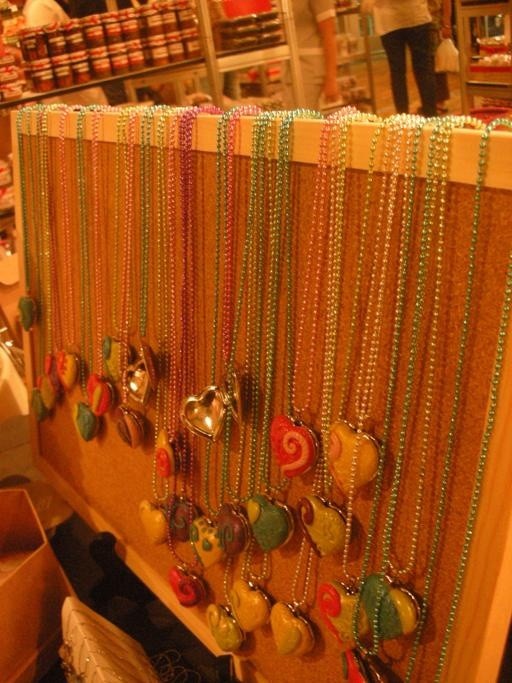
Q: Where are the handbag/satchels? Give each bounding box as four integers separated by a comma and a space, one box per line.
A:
317, 93, 344, 116
433, 31, 460, 74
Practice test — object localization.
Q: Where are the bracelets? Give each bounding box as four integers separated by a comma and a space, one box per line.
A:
441, 21, 452, 28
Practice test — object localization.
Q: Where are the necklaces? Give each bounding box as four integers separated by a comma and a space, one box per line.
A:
13, 99, 511, 682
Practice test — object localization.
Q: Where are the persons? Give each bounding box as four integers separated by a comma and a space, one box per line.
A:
206, 0, 245, 101
7, 0, 111, 107
424, 0, 456, 112
356, 0, 441, 118
289, 0, 344, 114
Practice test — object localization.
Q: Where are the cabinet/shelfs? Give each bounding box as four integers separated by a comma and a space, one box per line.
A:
0, 1, 512, 234
